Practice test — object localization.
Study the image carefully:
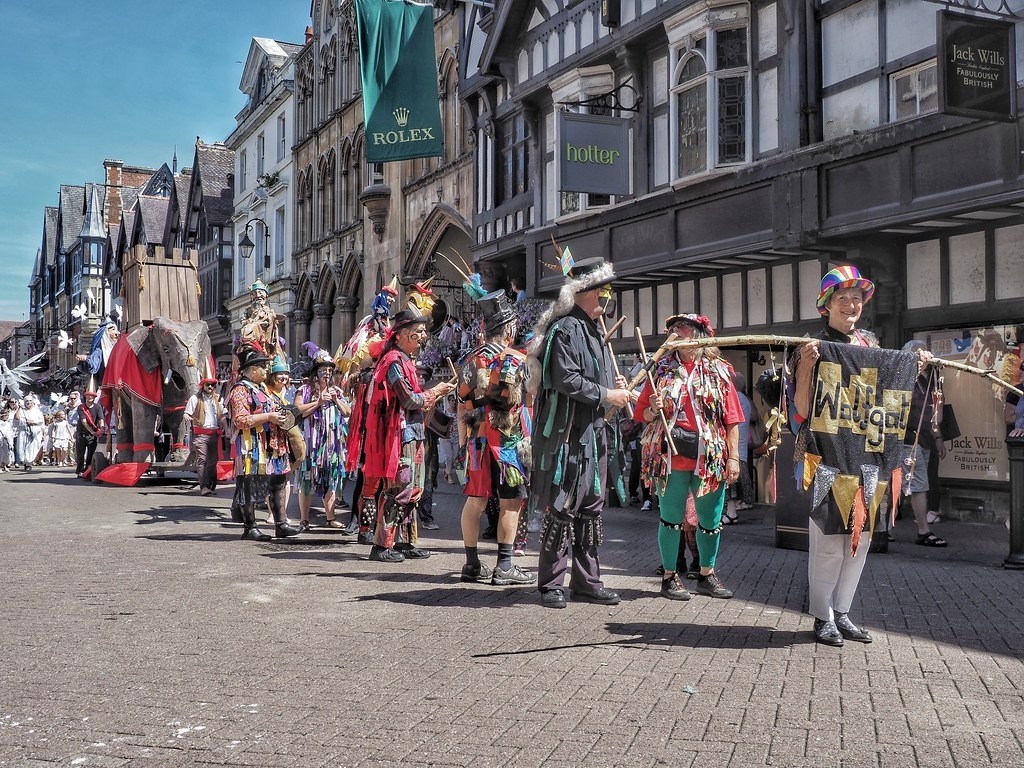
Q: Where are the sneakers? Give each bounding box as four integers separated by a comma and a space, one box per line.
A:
460, 517, 542, 585
357, 523, 441, 564
660, 572, 733, 601
629, 496, 653, 511
540, 586, 620, 609
656, 557, 699, 579
915, 511, 942, 524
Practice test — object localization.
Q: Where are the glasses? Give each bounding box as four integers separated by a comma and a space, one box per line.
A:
252, 363, 267, 368
600, 287, 615, 294
204, 382, 215, 387
668, 322, 692, 332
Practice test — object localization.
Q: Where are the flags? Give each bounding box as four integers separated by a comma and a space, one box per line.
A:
561, 248, 575, 276
353, 0, 445, 163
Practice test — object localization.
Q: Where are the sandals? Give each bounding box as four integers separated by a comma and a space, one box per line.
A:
721, 514, 739, 525
914, 532, 948, 547
735, 501, 753, 510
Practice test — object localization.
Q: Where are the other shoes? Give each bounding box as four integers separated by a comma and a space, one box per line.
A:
813, 616, 872, 646
245, 496, 360, 540
201, 487, 217, 495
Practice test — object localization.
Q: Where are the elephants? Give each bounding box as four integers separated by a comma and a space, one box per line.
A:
112, 316, 212, 463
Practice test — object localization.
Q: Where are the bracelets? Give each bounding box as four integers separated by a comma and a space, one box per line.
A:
729, 458, 740, 463
649, 407, 660, 416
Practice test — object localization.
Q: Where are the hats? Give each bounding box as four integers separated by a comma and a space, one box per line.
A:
816, 265, 875, 317
372, 234, 616, 336
1006, 324, 1024, 346
666, 311, 708, 335
85, 374, 98, 398
198, 341, 336, 389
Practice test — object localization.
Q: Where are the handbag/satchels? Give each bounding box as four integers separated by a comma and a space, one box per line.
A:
939, 403, 961, 441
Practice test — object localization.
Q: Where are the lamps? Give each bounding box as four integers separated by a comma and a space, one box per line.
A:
238, 218, 269, 272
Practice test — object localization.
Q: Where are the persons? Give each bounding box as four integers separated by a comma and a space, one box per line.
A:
1004, 359, 1024, 437
720, 372, 753, 525
0, 391, 82, 474
183, 378, 231, 496
786, 266, 935, 645
633, 313, 746, 600
1008, 395, 1024, 437
525, 256, 633, 608
76, 316, 121, 386
76, 392, 105, 478
888, 340, 947, 547
510, 278, 525, 301
243, 279, 286, 365
226, 288, 653, 585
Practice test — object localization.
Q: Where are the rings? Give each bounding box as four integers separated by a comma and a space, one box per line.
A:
812, 354, 815, 357
